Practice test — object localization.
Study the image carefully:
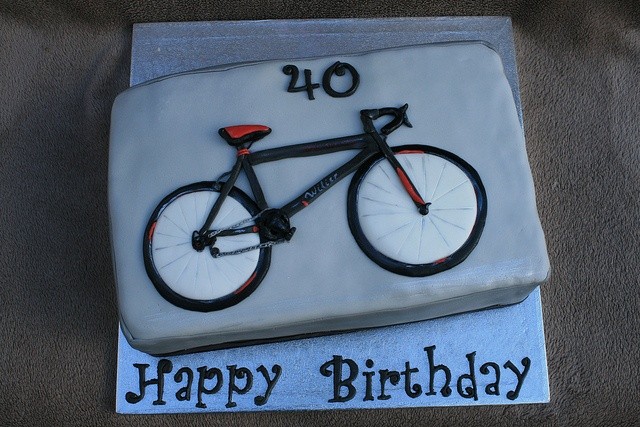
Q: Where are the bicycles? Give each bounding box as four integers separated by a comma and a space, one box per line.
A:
143, 104, 487, 312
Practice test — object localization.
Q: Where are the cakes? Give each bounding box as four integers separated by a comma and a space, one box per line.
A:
108, 40, 551, 357
